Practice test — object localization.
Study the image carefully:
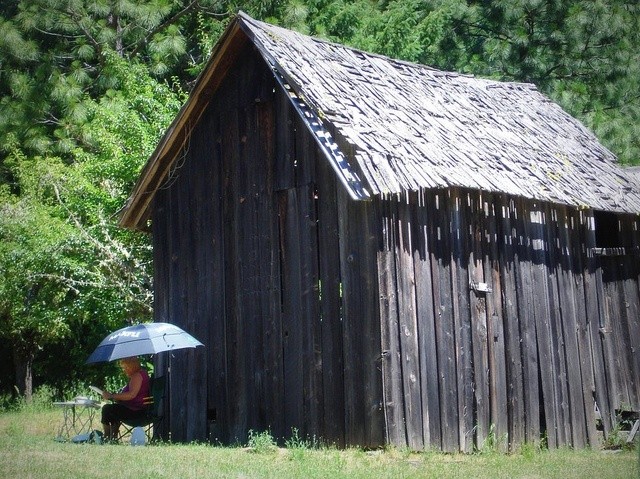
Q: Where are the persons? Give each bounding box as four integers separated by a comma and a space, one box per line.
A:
100, 356, 154, 445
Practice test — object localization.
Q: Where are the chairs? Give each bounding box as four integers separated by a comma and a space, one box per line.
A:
108, 369, 168, 443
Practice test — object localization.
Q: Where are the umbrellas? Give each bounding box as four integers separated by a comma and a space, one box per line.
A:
84, 322, 206, 367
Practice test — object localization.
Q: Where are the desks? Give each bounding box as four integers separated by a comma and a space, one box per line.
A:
53, 401, 103, 445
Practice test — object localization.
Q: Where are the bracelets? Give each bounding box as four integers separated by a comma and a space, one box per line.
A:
110, 393, 116, 398
